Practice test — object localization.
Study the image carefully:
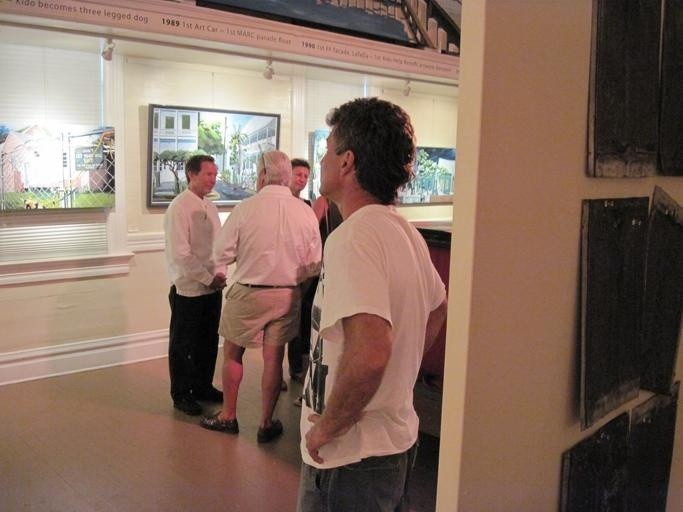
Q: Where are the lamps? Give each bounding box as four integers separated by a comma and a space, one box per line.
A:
262, 59, 274, 80
101, 39, 116, 63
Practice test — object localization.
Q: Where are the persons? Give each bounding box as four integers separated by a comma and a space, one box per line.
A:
297, 95, 448, 511
281, 158, 318, 391
160, 154, 229, 419
197, 151, 325, 443
292, 194, 346, 409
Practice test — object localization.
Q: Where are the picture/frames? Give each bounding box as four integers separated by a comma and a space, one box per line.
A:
147, 103, 280, 210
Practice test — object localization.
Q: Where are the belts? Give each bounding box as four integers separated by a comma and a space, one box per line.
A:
236, 282, 301, 289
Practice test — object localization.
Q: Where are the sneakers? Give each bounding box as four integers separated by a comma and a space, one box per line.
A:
257, 418, 282, 442
174, 393, 203, 416
199, 411, 239, 433
192, 387, 224, 404
280, 369, 307, 407
